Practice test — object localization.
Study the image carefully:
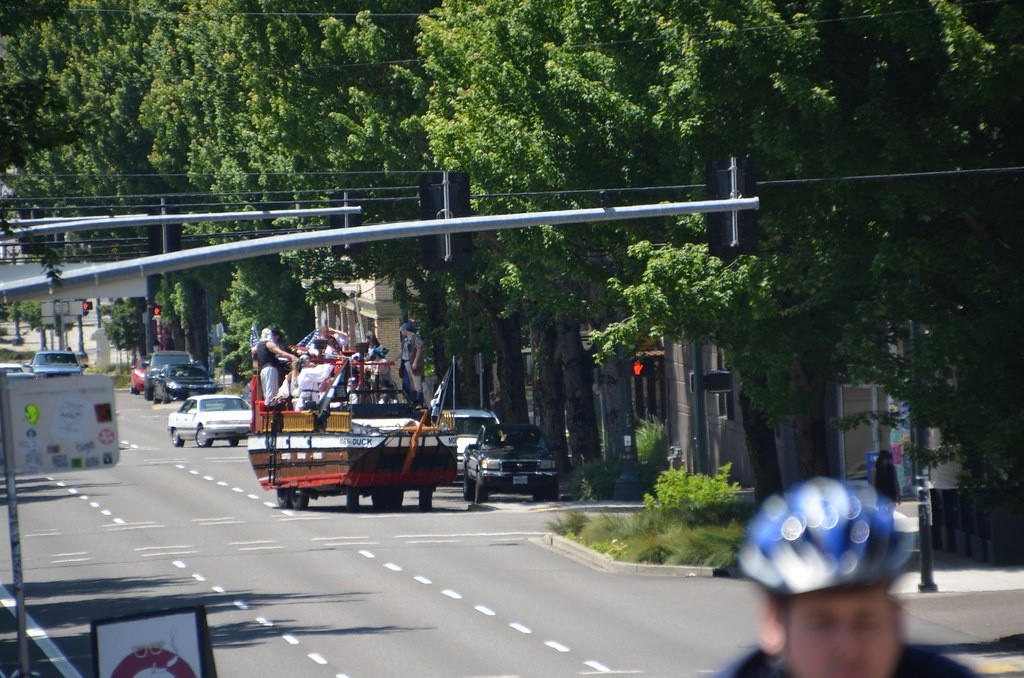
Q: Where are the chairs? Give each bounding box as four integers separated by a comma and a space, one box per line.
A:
506, 431, 522, 443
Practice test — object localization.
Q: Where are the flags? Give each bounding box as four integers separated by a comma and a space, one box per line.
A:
428, 365, 452, 427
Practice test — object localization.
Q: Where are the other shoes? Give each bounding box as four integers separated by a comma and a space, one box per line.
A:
265, 405, 281, 411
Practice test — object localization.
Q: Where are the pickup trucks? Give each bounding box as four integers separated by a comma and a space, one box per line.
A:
475, 424, 561, 504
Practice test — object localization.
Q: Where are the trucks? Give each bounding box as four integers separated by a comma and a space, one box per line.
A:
246, 327, 459, 514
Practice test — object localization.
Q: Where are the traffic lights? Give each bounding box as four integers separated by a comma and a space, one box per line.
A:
83, 301, 92, 310
631, 358, 651, 377
153, 306, 161, 315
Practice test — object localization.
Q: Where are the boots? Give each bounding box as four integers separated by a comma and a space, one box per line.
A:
415, 391, 424, 410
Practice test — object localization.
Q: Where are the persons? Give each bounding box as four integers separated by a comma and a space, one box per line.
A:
872, 450, 902, 521
253, 321, 428, 411
702, 473, 982, 678
929, 436, 974, 555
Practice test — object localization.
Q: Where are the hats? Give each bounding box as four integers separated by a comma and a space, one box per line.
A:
399, 322, 411, 331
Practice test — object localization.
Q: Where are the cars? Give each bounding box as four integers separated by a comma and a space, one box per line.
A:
153, 364, 217, 405
23, 351, 88, 377
129, 356, 151, 394
0, 364, 24, 374
143, 352, 201, 400
443, 409, 503, 473
167, 394, 254, 448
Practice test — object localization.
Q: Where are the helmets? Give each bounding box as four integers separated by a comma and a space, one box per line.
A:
735, 475, 924, 600
